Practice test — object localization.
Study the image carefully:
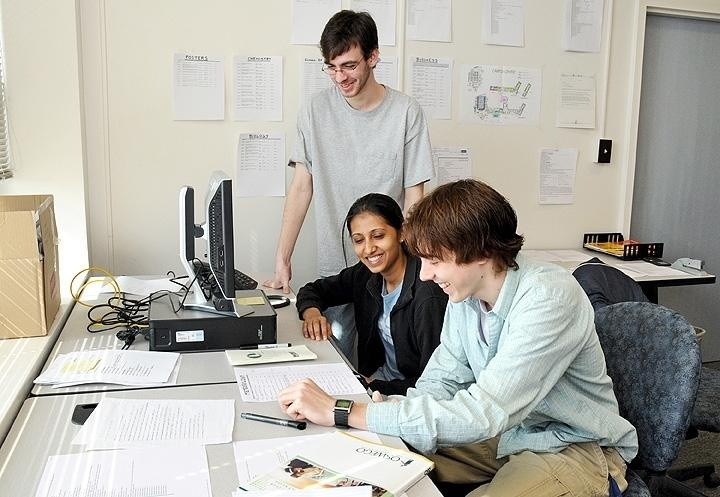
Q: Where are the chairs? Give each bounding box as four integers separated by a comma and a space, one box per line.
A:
595, 302, 702, 497
572, 264, 720, 497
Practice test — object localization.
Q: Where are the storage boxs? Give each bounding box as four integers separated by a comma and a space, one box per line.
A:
0, 195, 61, 339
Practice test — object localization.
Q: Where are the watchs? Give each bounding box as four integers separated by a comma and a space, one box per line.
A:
333, 398, 354, 428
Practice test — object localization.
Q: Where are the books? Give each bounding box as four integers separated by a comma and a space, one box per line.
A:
237, 428, 436, 496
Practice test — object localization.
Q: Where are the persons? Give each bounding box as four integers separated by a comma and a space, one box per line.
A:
262, 8, 435, 367
276, 175, 638, 497
295, 193, 448, 402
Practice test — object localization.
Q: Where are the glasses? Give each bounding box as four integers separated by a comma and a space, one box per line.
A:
320, 51, 369, 76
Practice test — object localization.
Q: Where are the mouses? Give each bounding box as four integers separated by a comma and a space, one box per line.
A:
267, 295, 290, 309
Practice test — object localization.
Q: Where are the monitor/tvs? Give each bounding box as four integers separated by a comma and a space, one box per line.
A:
176, 184, 208, 309
192, 170, 256, 319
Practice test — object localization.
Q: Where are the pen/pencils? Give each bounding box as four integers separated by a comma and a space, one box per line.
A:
240, 413, 307, 430
240, 343, 291, 350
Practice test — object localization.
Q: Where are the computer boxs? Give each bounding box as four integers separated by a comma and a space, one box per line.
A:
148, 289, 277, 354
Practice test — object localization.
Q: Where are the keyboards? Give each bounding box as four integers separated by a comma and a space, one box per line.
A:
234, 264, 258, 293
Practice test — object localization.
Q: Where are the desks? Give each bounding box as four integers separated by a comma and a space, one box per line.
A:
0, 378, 445, 497
30, 276, 362, 396
519, 248, 716, 304
